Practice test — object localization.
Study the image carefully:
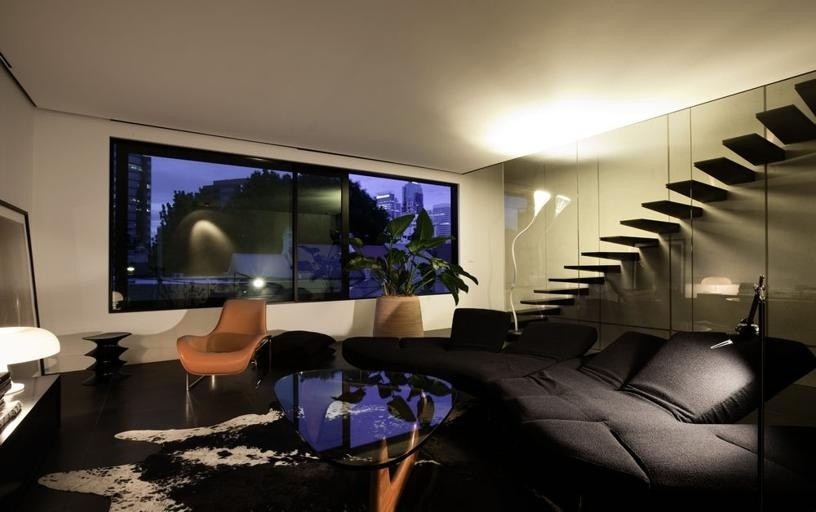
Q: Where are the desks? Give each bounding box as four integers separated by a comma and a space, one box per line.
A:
0, 374, 61, 511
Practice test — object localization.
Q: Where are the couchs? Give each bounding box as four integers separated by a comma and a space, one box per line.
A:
342, 308, 816, 512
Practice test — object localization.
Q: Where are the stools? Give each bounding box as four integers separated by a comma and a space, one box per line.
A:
254, 329, 338, 386
81, 332, 131, 387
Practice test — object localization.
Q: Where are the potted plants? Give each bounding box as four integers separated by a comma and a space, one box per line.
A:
339, 208, 478, 338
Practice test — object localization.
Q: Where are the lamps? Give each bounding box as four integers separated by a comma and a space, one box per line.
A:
536, 195, 570, 273
0, 327, 61, 394
506, 190, 551, 342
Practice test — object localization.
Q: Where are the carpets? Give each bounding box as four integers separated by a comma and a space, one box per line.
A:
38, 399, 563, 511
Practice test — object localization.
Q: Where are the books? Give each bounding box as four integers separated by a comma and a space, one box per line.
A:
0, 370, 21, 430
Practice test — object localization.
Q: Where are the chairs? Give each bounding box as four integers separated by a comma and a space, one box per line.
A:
176, 300, 272, 392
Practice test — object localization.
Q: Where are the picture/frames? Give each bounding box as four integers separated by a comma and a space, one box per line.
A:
0, 198, 44, 377
632, 239, 685, 301
